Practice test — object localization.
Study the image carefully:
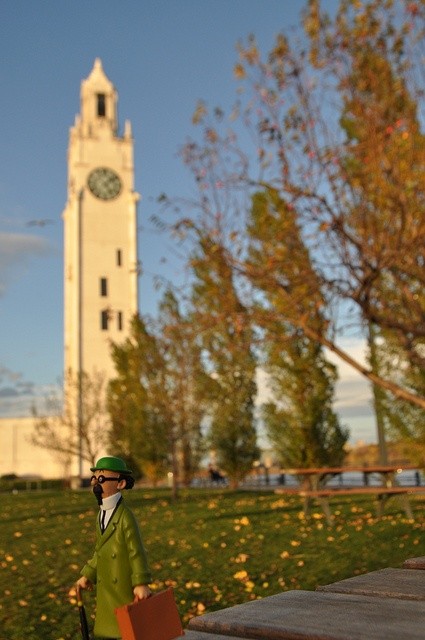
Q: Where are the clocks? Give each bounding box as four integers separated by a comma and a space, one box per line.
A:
88, 167, 123, 201
